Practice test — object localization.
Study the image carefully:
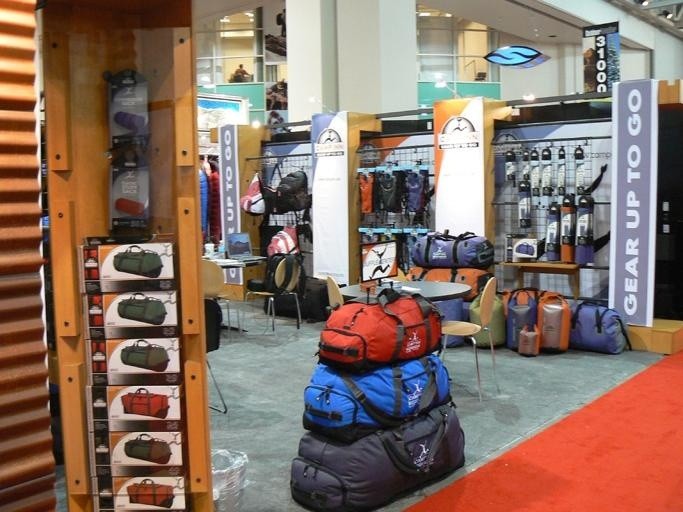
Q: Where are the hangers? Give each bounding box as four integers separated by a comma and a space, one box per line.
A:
199, 154, 219, 172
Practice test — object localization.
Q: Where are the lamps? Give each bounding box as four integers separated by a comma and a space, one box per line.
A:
634, 0, 649, 6
309, 95, 334, 113
657, 9, 673, 19
434, 76, 458, 98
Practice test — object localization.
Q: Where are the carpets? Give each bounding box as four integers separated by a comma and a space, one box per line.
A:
406, 350, 683, 512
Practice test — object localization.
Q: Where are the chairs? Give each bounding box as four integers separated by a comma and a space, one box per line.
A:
234, 74, 243, 83
242, 257, 301, 332
475, 72, 486, 82
326, 276, 343, 313
201, 260, 239, 346
442, 276, 499, 402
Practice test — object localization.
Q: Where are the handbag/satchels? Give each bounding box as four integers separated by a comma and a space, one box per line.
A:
114, 246, 173, 507
290, 288, 464, 512
405, 230, 494, 301
470, 287, 631, 354
241, 164, 312, 215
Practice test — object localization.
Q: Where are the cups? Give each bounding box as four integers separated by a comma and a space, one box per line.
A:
204, 243, 214, 254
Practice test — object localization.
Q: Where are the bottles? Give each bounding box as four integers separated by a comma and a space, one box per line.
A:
218, 240, 226, 258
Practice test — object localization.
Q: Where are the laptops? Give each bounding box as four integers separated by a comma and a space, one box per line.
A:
222, 233, 268, 261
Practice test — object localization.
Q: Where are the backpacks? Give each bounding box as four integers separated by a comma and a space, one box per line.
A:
268, 226, 300, 256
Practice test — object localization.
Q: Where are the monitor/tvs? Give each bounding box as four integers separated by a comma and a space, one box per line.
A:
358, 240, 399, 283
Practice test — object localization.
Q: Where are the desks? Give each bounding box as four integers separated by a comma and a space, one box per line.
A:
340, 282, 472, 302
212, 255, 265, 269
229, 73, 253, 82
